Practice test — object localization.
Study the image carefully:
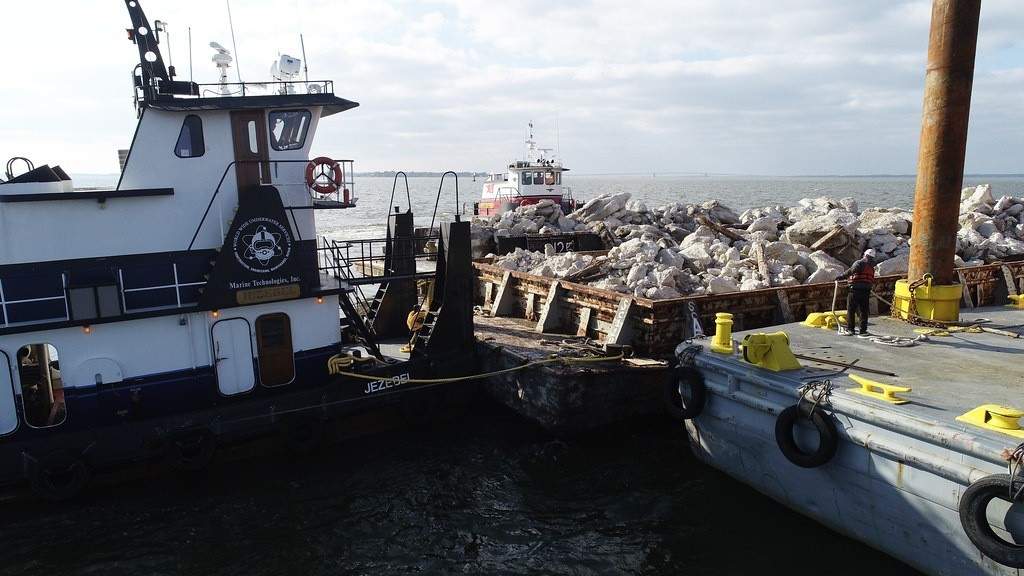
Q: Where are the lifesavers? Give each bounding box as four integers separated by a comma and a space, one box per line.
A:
958, 471, 1024, 570
305, 156, 344, 193
664, 367, 705, 420
774, 403, 838, 470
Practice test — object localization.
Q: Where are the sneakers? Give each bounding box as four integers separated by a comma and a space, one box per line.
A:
860, 330, 871, 336
846, 328, 856, 335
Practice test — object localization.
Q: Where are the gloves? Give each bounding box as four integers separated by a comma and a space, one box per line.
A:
834, 275, 844, 280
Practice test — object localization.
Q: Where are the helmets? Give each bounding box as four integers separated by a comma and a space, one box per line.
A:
864, 249, 876, 258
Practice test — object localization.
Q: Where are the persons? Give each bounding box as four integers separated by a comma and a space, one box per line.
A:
834, 249, 876, 336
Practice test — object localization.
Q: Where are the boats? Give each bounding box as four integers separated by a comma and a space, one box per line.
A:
1, 0, 367, 489
466, 121, 582, 216
674, 1, 1023, 576
